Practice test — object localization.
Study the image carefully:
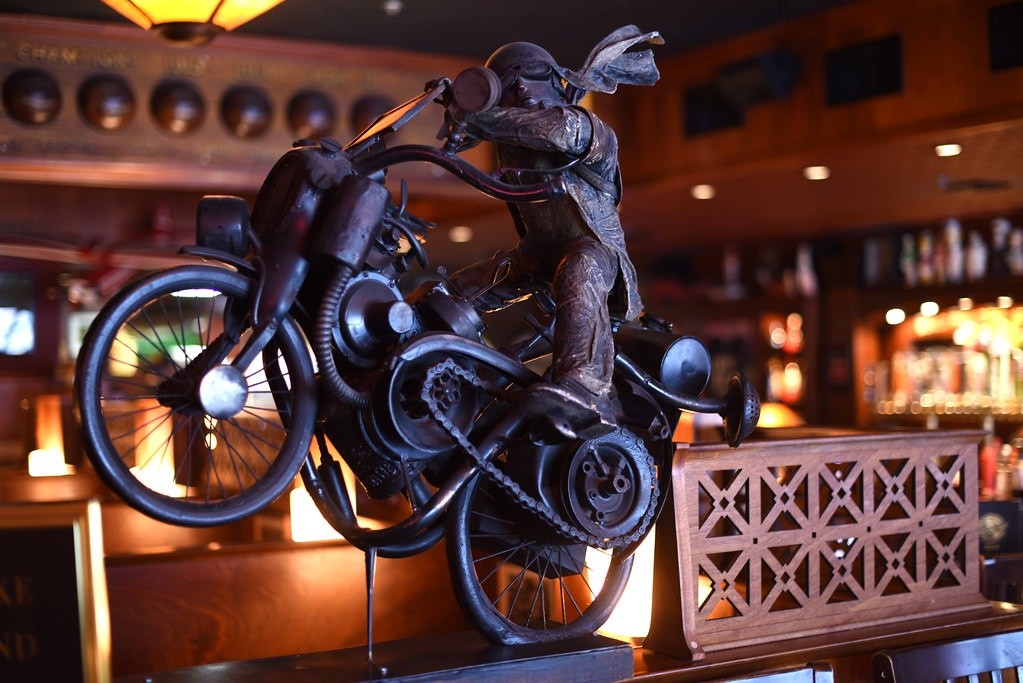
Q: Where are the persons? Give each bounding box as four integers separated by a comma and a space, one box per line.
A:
424, 42, 646, 440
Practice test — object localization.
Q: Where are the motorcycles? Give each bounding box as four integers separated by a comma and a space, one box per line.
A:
74, 74, 764, 650
